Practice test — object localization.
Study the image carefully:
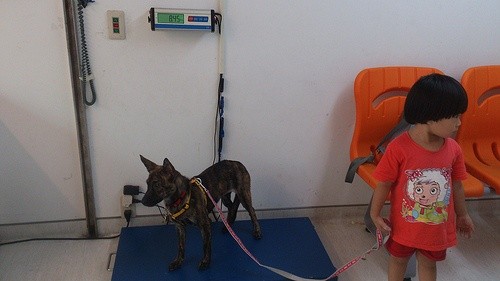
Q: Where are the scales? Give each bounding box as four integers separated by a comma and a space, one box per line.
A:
110, 7, 338, 281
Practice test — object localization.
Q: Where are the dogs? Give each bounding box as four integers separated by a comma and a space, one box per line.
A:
139, 153, 264, 274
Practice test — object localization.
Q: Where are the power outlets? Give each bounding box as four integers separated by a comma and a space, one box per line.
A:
120, 194, 136, 219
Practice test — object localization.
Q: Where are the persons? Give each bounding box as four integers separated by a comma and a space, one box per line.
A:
369, 73, 475, 281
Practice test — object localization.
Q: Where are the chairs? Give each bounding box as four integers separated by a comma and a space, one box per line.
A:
350, 66, 483, 239
457, 65, 500, 191
